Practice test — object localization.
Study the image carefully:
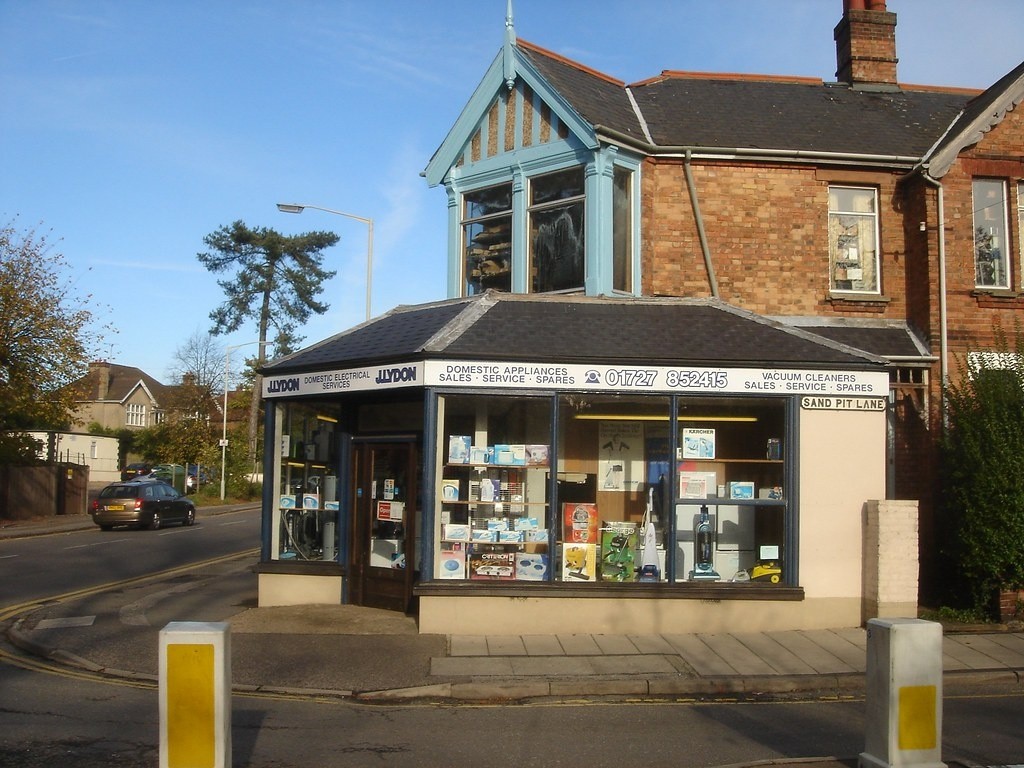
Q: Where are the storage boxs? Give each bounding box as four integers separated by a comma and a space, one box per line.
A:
281, 494, 296, 510
302, 493, 320, 510
438, 550, 549, 582
441, 478, 460, 503
562, 501, 639, 584
681, 427, 716, 461
834, 266, 864, 282
440, 516, 549, 545
447, 435, 551, 467
679, 470, 717, 500
725, 481, 755, 501
324, 500, 340, 512
848, 247, 857, 260
481, 478, 500, 505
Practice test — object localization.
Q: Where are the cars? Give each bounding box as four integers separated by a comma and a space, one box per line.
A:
121, 463, 152, 483
308, 476, 323, 489
92, 481, 196, 530
282, 478, 311, 492
129, 468, 204, 492
151, 464, 185, 473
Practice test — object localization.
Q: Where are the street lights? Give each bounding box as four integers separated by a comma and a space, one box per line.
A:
221, 341, 276, 500
277, 204, 373, 323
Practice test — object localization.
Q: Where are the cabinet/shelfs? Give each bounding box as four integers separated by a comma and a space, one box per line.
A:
437, 463, 549, 583
